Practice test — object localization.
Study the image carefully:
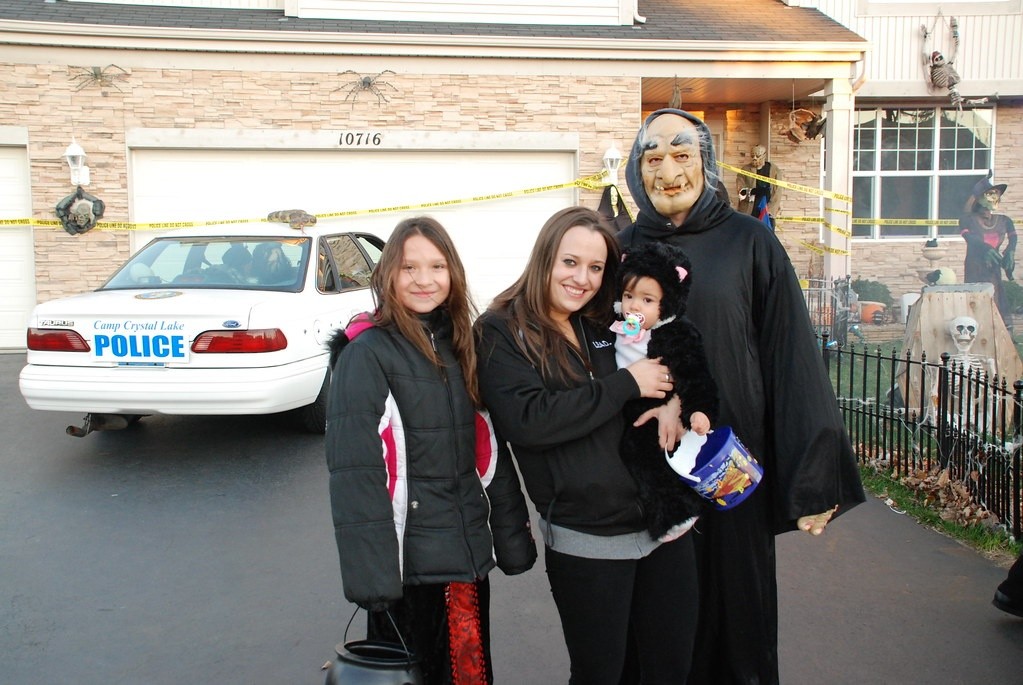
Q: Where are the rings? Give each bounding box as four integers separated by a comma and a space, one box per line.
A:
665, 374, 670, 382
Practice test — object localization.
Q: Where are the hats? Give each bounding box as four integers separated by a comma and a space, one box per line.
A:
971, 177, 1007, 211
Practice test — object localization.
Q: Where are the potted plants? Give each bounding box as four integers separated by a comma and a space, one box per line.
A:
849, 278, 894, 324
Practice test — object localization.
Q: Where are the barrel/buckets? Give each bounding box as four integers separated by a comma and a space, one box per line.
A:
665, 425, 764, 511
325, 603, 427, 685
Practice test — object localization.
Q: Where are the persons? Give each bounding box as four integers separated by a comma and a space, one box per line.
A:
734, 145, 784, 228
957, 178, 1020, 343
991, 556, 1022, 618
324, 216, 538, 685
610, 109, 866, 685
607, 239, 718, 544
474, 207, 708, 685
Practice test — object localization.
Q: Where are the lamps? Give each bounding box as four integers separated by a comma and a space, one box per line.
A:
602, 144, 622, 187
61, 136, 92, 188
